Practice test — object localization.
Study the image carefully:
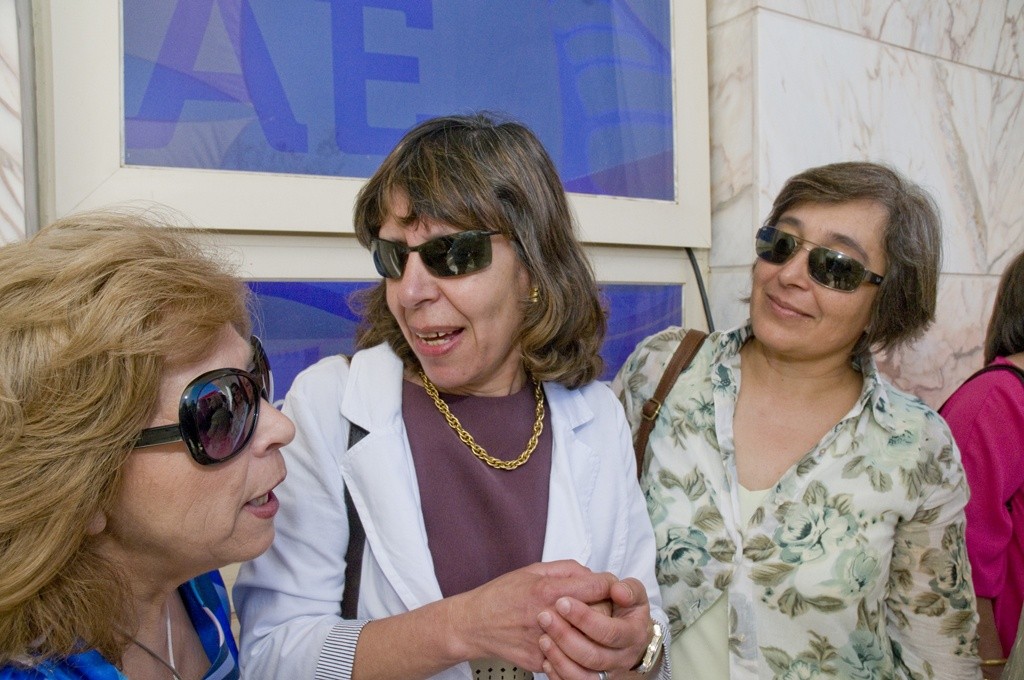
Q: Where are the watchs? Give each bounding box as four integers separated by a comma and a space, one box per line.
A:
633, 624, 663, 675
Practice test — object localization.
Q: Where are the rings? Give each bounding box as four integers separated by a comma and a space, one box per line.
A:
598, 671, 608, 680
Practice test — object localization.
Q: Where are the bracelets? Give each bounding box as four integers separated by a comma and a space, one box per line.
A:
980, 659, 1008, 665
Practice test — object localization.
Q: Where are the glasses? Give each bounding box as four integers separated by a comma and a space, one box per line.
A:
757, 225, 885, 293
121, 335, 273, 466
373, 230, 507, 280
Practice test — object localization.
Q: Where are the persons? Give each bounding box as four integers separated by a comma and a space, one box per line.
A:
938, 254, 1024, 680
0, 208, 295, 680
610, 162, 980, 680
232, 116, 670, 680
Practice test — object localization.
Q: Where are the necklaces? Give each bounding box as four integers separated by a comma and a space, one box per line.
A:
108, 604, 183, 680
416, 369, 544, 471
